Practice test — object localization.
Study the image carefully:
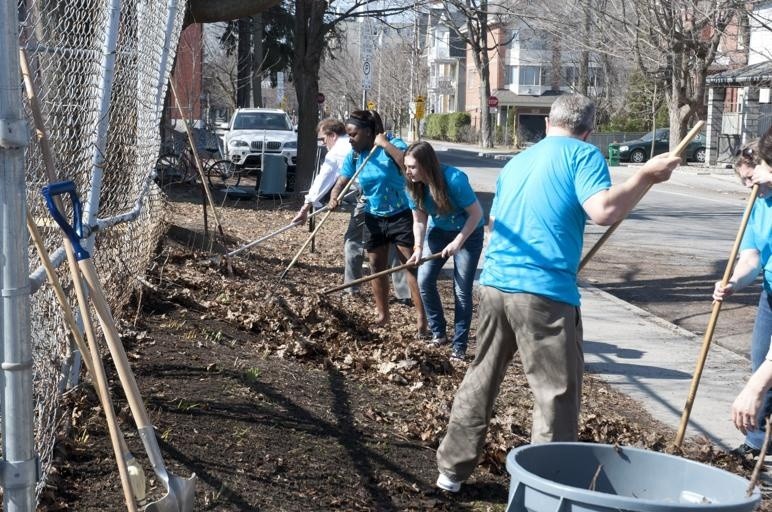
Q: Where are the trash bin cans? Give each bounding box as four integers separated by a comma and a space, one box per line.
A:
608, 143, 620, 166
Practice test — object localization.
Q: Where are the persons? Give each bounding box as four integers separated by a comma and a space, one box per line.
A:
712, 137, 772, 457
437, 89, 683, 497
729, 127, 772, 436
328, 108, 432, 339
289, 117, 415, 307
405, 140, 486, 362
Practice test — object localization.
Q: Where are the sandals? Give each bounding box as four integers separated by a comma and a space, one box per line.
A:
428, 335, 448, 348
449, 351, 465, 361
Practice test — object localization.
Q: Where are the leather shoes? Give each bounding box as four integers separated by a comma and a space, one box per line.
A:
400, 298, 411, 306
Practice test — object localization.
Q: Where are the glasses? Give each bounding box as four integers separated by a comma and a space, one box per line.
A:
737, 148, 754, 156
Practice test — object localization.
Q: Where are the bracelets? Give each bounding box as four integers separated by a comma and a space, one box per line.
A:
412, 243, 425, 251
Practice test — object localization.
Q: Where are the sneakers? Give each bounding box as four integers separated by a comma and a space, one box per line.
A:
729, 443, 772, 455
436, 471, 461, 492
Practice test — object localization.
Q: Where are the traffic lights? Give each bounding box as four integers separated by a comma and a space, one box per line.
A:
318, 93, 325, 103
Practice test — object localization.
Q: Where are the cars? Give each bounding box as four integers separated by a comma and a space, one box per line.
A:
215, 108, 298, 170
619, 127, 706, 163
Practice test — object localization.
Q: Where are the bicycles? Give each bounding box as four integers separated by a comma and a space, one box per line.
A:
156, 131, 240, 190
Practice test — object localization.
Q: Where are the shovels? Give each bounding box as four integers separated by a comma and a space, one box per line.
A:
41, 181, 196, 510
